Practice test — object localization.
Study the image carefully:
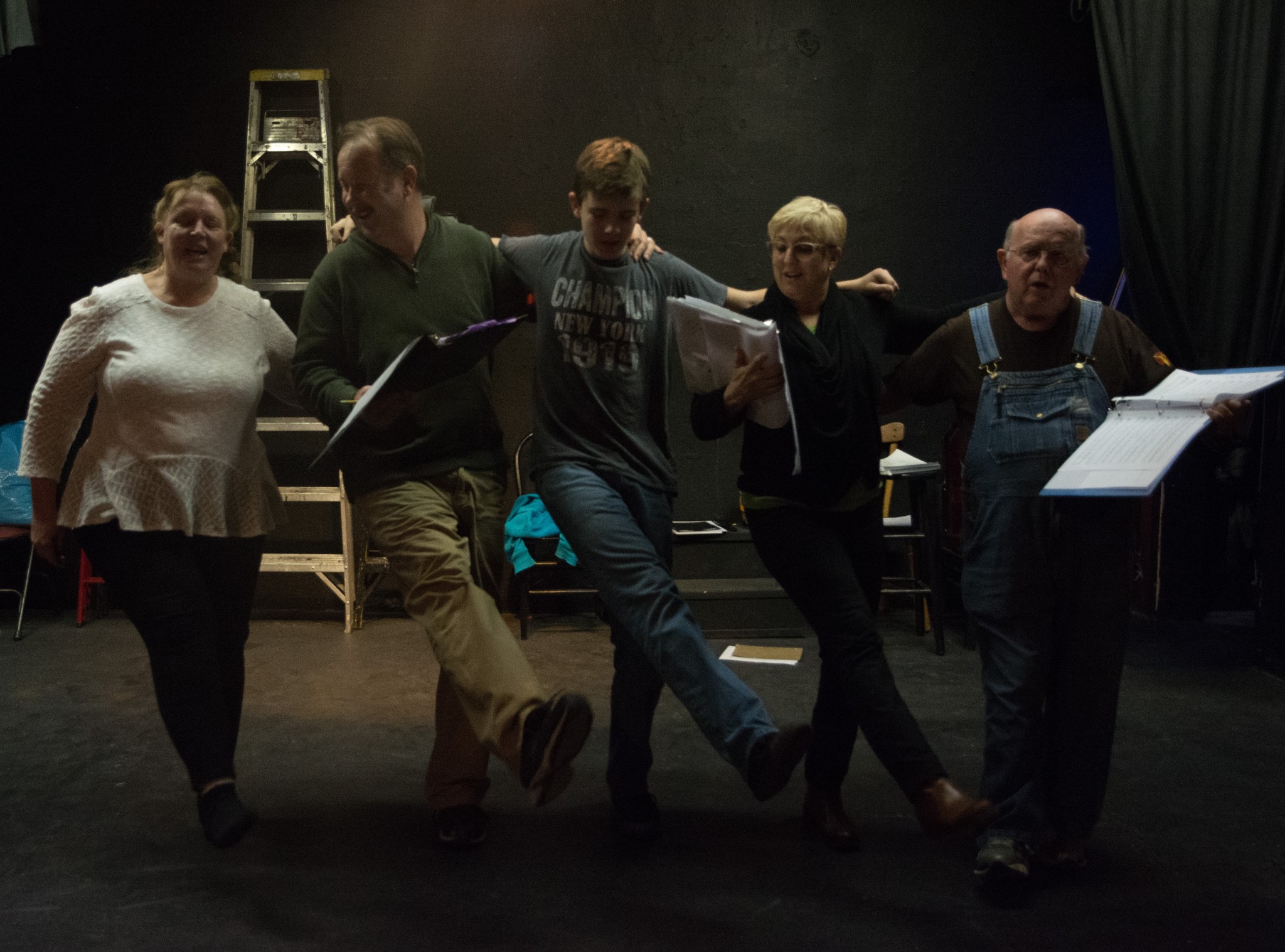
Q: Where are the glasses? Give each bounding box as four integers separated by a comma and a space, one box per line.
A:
766, 241, 834, 261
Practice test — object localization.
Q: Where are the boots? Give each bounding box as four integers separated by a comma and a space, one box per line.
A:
918, 780, 996, 842
803, 779, 865, 841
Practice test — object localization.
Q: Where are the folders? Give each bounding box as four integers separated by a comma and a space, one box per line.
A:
1037, 368, 1285, 498
303, 315, 525, 466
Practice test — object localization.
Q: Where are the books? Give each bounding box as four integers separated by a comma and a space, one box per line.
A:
669, 294, 802, 476
307, 309, 523, 467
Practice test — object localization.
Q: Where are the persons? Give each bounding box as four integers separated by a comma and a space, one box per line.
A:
16, 170, 306, 846
292, 116, 665, 849
324, 137, 899, 845
681, 196, 995, 853
887, 206, 1256, 908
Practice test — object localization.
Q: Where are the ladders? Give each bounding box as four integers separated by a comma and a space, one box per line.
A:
238, 66, 366, 632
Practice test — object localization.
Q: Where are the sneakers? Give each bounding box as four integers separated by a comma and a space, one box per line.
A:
751, 723, 812, 801
521, 692, 593, 809
977, 834, 1034, 878
611, 795, 667, 845
1043, 832, 1090, 869
435, 808, 490, 851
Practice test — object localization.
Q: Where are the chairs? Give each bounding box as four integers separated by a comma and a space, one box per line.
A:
0, 420, 65, 639
879, 421, 937, 628
511, 429, 612, 639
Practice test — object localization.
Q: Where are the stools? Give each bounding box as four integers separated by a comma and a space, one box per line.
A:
880, 459, 949, 657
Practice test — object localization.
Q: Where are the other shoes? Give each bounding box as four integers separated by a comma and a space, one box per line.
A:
201, 785, 257, 848
